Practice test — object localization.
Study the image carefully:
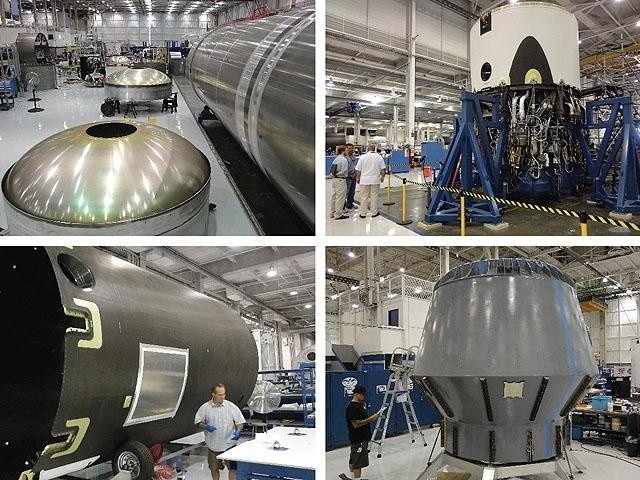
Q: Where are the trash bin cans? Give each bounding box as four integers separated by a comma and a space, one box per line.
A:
625, 436, 639, 457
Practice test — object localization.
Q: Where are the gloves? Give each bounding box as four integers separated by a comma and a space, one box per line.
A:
205, 424, 218, 433
231, 431, 241, 440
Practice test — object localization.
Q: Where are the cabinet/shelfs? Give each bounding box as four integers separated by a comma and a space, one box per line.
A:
572, 405, 639, 440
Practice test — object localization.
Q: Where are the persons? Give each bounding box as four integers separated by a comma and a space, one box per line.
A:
194, 384, 246, 480
339, 385, 382, 480
355, 144, 386, 218
330, 143, 357, 220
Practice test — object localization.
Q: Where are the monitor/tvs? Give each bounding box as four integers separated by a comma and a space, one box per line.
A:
603, 376, 632, 399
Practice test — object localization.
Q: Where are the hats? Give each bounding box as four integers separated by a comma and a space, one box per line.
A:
352, 386, 366, 393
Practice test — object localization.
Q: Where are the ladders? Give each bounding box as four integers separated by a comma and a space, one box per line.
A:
366, 373, 427, 459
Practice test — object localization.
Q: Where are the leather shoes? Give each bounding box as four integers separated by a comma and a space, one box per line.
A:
330, 204, 380, 219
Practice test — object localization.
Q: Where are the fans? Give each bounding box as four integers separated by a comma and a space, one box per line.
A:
247, 380, 281, 432
25, 72, 45, 112
